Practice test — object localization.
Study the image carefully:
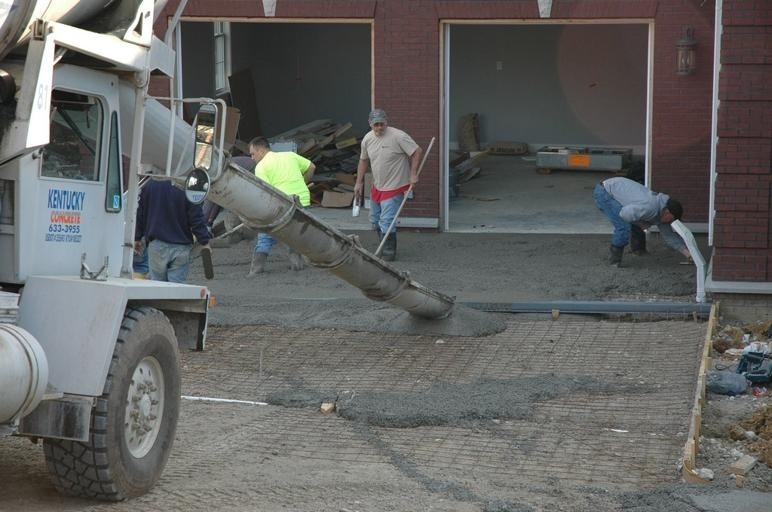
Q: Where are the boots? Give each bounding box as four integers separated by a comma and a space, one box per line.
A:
377, 230, 396, 260
245, 252, 267, 278
630, 234, 646, 252
608, 244, 622, 268
288, 250, 303, 270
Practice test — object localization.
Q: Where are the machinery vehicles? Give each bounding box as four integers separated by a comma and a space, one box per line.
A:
0, 0, 227, 503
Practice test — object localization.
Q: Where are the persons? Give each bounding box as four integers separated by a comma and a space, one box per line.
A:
134, 181, 213, 282
248, 136, 316, 274
592, 176, 691, 268
354, 109, 423, 262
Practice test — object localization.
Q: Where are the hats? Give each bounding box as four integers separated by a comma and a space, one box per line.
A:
368, 110, 388, 126
666, 201, 682, 223
